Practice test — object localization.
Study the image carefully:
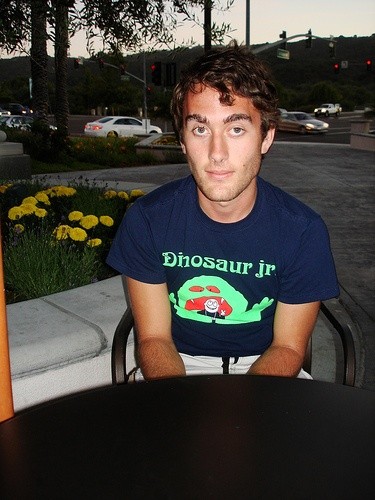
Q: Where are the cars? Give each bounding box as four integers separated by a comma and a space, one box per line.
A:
85, 116, 162, 136
0, 103, 57, 130
277, 108, 329, 134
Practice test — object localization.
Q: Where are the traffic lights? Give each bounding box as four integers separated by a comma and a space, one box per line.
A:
306, 29, 312, 49
279, 31, 287, 50
98, 52, 105, 68
332, 62, 339, 71
328, 41, 336, 58
120, 64, 126, 74
151, 62, 165, 87
147, 87, 151, 92
366, 59, 373, 71
168, 62, 178, 87
74, 59, 80, 69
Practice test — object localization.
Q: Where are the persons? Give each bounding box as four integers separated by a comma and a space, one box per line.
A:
106, 40, 342, 381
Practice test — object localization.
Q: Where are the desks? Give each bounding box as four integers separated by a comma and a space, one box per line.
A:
0, 373, 375, 500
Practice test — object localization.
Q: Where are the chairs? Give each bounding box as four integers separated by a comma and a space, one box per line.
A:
110, 295, 358, 387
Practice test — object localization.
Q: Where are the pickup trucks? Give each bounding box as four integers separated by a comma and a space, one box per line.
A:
314, 103, 342, 117
